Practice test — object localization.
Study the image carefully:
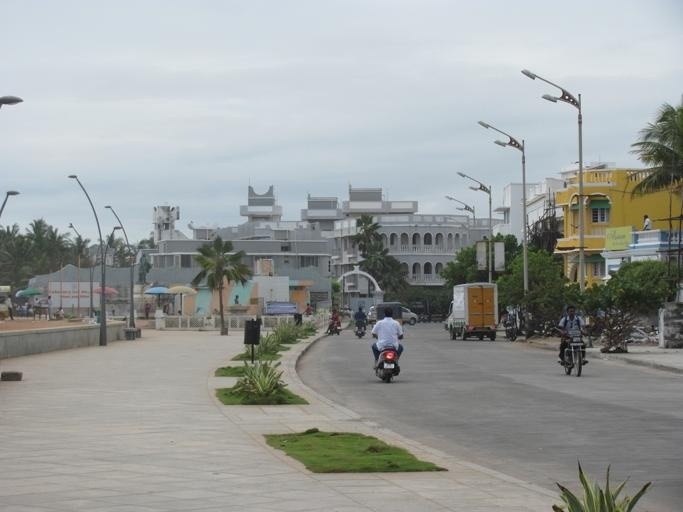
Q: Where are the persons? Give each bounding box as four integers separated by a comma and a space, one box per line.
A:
369, 307, 404, 365
323, 309, 339, 334
144, 304, 150, 320
300, 303, 312, 315
640, 214, 651, 231
11, 298, 39, 317
353, 306, 366, 330
46, 295, 53, 320
554, 305, 591, 363
233, 294, 239, 304
6, 294, 14, 320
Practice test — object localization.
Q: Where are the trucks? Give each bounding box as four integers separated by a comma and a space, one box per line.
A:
447, 283, 499, 341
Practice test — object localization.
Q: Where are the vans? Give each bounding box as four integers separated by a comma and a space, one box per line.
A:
367, 306, 418, 325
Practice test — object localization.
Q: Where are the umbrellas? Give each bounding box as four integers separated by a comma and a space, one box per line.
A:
143, 286, 176, 308
92, 286, 119, 295
14, 288, 42, 298
166, 285, 198, 315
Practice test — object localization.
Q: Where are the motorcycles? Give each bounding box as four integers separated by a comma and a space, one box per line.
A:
375, 343, 400, 382
356, 320, 366, 337
328, 318, 340, 335
559, 329, 585, 376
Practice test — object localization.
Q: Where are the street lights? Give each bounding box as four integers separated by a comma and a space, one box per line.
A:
66, 175, 137, 347
457, 170, 493, 283
477, 120, 527, 296
444, 194, 477, 247
522, 69, 585, 293
